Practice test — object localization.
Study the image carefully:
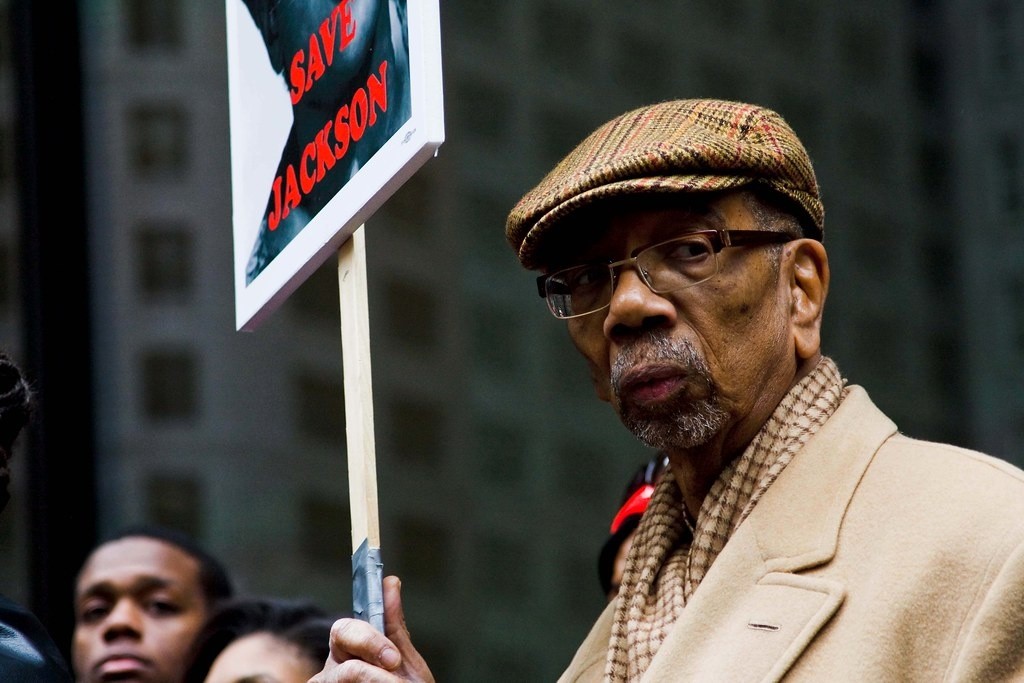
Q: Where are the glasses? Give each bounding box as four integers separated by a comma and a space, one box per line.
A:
537, 228, 799, 319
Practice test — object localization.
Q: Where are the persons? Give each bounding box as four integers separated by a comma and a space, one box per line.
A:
595, 454, 674, 603
310, 98, 1023, 683
0, 347, 338, 683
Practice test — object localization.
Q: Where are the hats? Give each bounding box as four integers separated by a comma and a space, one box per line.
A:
506, 99, 824, 269
